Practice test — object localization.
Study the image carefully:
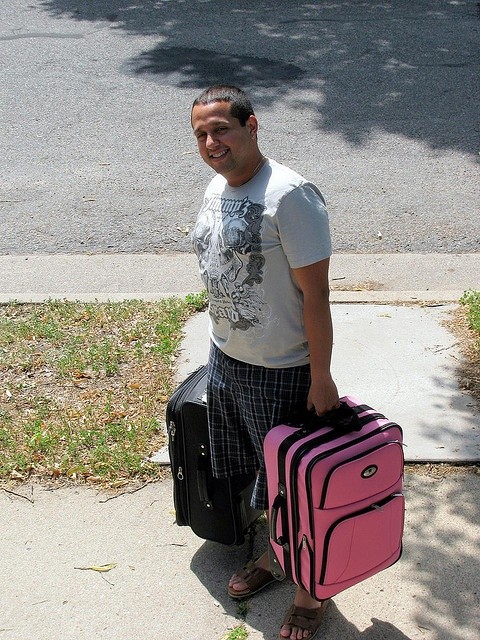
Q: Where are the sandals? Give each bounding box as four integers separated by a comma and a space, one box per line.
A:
227, 548, 286, 600
278, 598, 330, 640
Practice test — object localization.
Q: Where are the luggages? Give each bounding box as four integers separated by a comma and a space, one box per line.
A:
262, 396, 406, 602
166, 362, 265, 548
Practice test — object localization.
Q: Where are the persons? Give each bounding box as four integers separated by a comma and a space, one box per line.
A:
190, 85, 339, 640
